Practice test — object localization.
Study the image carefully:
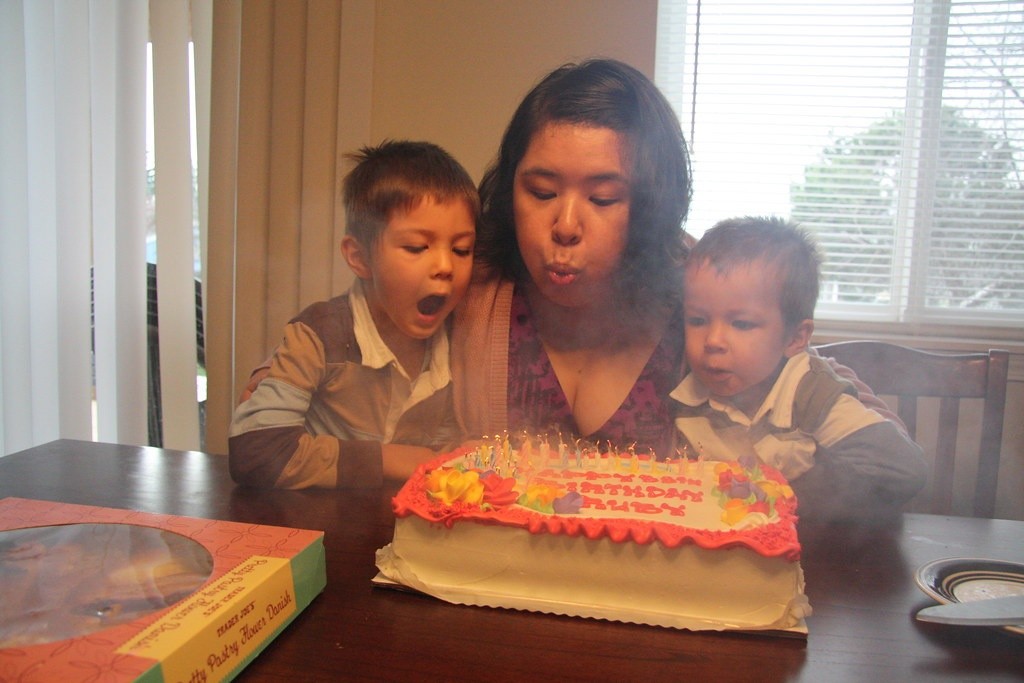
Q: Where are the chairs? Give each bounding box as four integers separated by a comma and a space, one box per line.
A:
815, 338, 1011, 519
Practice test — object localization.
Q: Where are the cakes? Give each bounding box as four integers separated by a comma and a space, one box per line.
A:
390, 430, 805, 623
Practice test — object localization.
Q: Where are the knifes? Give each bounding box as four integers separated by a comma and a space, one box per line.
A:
916, 594, 1024, 627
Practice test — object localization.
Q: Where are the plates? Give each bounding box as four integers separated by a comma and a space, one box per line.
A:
913, 555, 1024, 643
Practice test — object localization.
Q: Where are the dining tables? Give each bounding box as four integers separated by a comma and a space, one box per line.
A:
0, 440, 1023, 683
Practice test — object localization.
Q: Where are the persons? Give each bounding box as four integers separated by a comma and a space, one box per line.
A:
226, 138, 483, 494
242, 56, 912, 462
667, 216, 927, 538
